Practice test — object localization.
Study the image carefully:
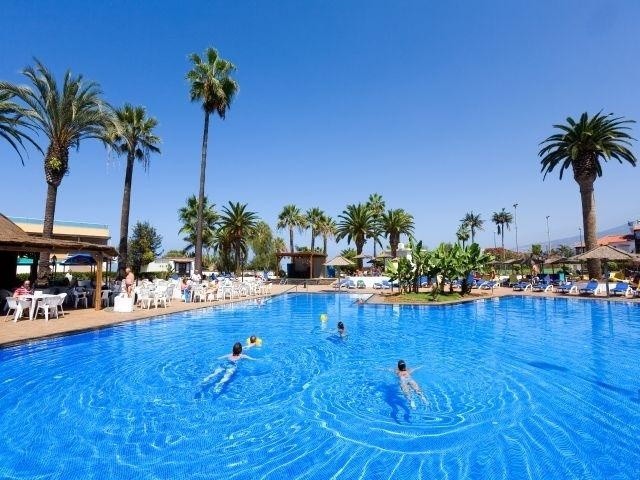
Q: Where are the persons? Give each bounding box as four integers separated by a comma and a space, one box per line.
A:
246, 336, 262, 347
12, 267, 134, 316
203, 341, 259, 384
51, 255, 58, 268
384, 361, 430, 410
337, 322, 346, 338
474, 272, 481, 282
181, 272, 218, 295
355, 267, 382, 276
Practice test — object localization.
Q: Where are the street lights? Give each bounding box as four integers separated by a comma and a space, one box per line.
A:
545, 215, 551, 253
579, 227, 583, 253
513, 203, 519, 252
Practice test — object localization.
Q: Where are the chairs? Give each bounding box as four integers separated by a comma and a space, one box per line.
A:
333, 279, 366, 289
610, 282, 633, 297
452, 277, 500, 290
1, 274, 273, 324
373, 281, 390, 289
513, 276, 600, 296
416, 277, 434, 287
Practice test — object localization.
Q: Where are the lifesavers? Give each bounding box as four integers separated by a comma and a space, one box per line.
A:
248, 336, 262, 345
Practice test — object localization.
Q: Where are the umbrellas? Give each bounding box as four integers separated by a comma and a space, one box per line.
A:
321, 256, 357, 289
486, 244, 633, 298
60, 254, 115, 274
